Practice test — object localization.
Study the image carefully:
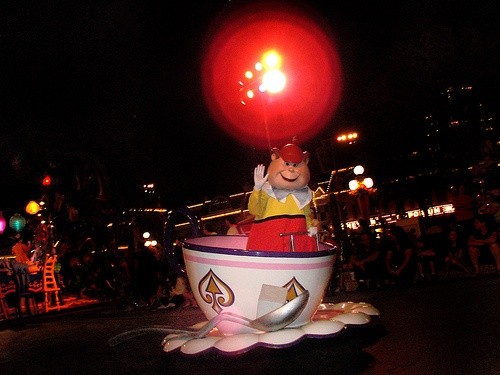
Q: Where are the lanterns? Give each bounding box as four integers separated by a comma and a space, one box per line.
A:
26, 200, 40, 216
0, 218, 8, 235
41, 176, 50, 186
8, 214, 26, 230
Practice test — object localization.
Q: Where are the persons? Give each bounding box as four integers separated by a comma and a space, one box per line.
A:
0, 232, 243, 308
310, 188, 500, 293
244, 142, 320, 252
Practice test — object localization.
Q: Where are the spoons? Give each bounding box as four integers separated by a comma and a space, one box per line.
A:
108, 290, 309, 347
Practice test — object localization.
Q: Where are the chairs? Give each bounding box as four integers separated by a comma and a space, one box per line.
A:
8, 264, 39, 319
28, 255, 61, 313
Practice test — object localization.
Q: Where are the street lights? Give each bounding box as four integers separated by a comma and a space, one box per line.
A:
349, 165, 374, 226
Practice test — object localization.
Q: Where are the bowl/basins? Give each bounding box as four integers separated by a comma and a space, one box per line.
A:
182, 235, 337, 336
